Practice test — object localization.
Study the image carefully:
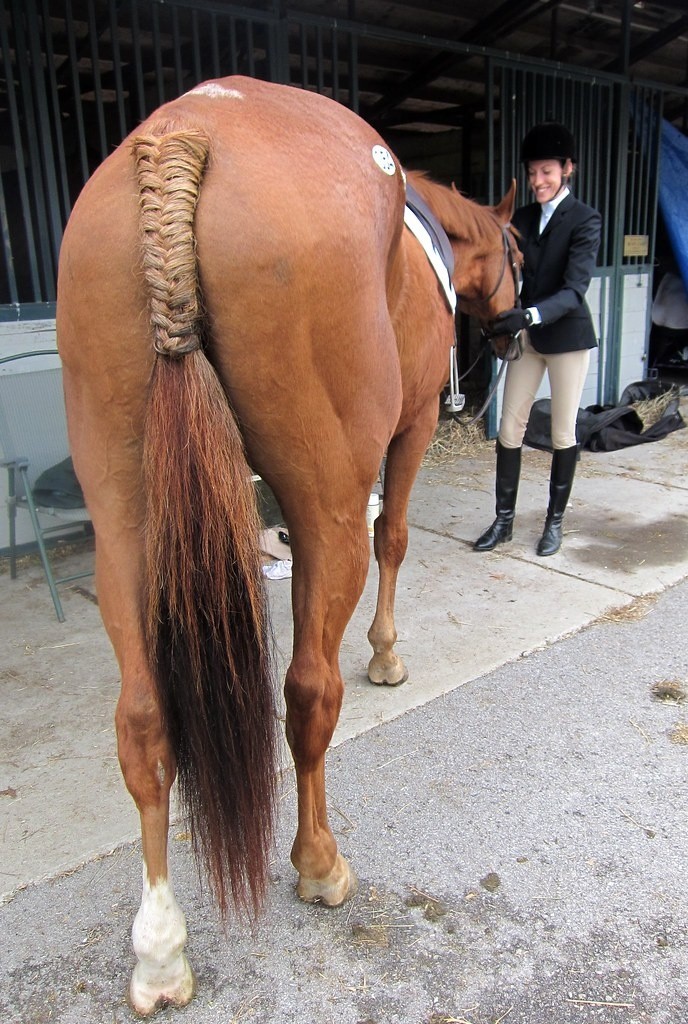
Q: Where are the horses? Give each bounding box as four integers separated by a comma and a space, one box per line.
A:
56, 74, 524, 1013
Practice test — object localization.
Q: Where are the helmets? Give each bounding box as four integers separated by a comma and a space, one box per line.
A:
520, 123, 578, 164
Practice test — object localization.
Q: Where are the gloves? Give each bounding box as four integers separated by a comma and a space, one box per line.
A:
488, 309, 531, 340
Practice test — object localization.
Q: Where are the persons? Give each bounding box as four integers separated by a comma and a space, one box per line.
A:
472, 122, 601, 557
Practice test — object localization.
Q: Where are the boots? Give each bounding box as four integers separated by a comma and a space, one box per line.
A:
537, 446, 578, 556
474, 438, 522, 551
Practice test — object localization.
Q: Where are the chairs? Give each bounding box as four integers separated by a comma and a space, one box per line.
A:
1, 349, 98, 621
